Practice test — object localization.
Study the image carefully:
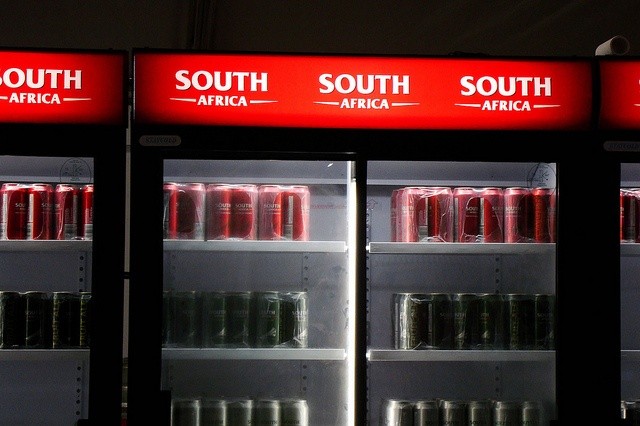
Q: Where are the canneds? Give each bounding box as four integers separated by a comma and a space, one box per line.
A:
29, 182, 54, 240
397, 186, 403, 240
230, 397, 255, 424
229, 290, 252, 346
26, 290, 51, 347
285, 182, 310, 240
173, 396, 200, 425
469, 399, 492, 425
164, 179, 178, 239
404, 291, 432, 349
415, 398, 437, 424
83, 185, 93, 234
621, 396, 640, 420
507, 292, 535, 349
283, 289, 311, 348
531, 185, 555, 242
389, 186, 398, 241
506, 186, 530, 242
379, 396, 411, 424
202, 290, 226, 346
494, 399, 517, 425
181, 287, 201, 348
634, 191, 639, 242
430, 186, 452, 240
2, 181, 28, 240
439, 399, 467, 425
455, 187, 481, 243
455, 293, 479, 350
202, 396, 227, 425
260, 186, 283, 240
522, 397, 548, 425
80, 291, 91, 347
182, 183, 206, 239
284, 400, 309, 425
209, 183, 232, 237
483, 184, 505, 242
537, 292, 555, 348
391, 291, 401, 349
431, 291, 454, 347
161, 289, 179, 343
398, 297, 407, 347
231, 185, 257, 240
56, 183, 79, 239
256, 402, 283, 426
54, 288, 79, 347
1, 289, 23, 347
621, 188, 636, 241
479, 291, 505, 350
254, 287, 281, 347
403, 185, 428, 240
